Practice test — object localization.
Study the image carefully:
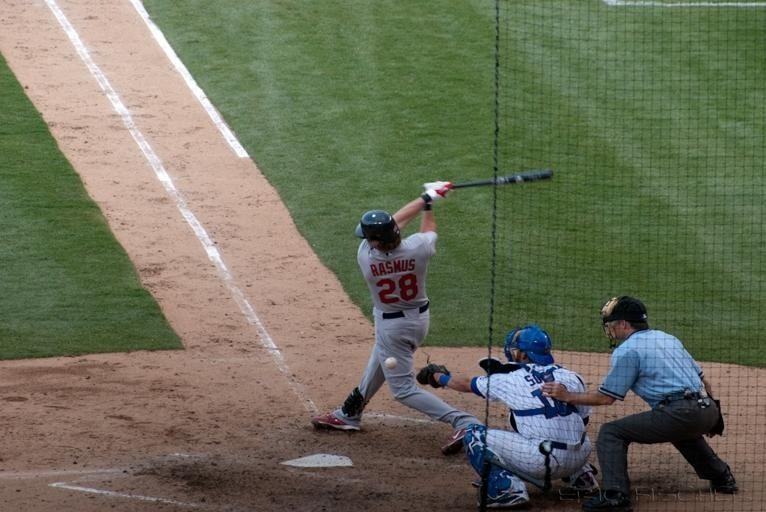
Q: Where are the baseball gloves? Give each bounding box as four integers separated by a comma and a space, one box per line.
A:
416, 364, 450, 388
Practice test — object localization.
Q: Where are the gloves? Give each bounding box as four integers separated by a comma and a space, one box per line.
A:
383, 303, 430, 321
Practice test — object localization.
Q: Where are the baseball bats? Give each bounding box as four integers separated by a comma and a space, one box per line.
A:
449, 170, 553, 189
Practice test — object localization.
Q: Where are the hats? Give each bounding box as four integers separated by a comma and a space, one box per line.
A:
354, 209, 400, 243
506, 325, 554, 366
601, 295, 650, 332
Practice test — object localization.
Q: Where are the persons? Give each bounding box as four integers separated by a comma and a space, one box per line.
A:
540, 295, 738, 512
311, 180, 485, 456
417, 325, 601, 511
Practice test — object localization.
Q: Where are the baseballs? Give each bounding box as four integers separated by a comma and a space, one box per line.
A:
384, 357, 398, 369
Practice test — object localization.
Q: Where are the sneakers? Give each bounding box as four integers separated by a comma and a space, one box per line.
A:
439, 428, 468, 454
475, 486, 529, 508
582, 490, 633, 511
311, 406, 361, 432
547, 484, 603, 500
710, 472, 739, 493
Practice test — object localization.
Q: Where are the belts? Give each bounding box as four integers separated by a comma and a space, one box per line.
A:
551, 432, 587, 450
661, 392, 709, 406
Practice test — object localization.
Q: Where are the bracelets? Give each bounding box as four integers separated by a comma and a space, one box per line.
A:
420, 193, 432, 203
423, 202, 431, 211
440, 375, 451, 385
